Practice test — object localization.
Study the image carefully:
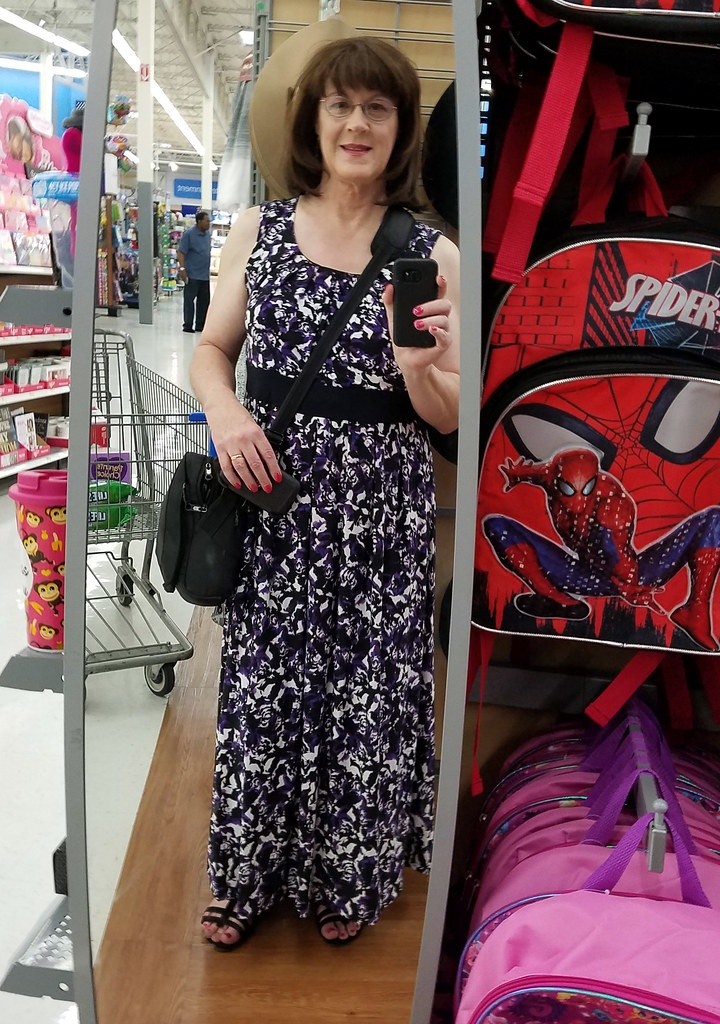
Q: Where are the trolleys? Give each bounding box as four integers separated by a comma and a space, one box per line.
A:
82, 329, 210, 701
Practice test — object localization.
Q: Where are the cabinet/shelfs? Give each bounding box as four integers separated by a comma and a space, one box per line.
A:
0, 263, 72, 481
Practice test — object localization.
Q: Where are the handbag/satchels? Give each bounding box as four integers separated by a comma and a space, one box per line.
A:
156, 451, 252, 606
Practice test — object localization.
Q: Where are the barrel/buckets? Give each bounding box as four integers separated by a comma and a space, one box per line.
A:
9, 469, 67, 653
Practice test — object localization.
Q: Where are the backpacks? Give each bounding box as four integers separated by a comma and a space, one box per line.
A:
447, 0, 719, 1024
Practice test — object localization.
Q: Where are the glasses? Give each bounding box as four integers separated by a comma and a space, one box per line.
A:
317, 95, 398, 122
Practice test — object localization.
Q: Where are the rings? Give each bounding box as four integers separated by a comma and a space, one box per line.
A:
231, 455, 242, 460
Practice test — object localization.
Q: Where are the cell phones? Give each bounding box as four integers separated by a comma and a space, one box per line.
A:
393, 260, 439, 348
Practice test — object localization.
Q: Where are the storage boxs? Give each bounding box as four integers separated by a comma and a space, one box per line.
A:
89, 453, 132, 486
0, 435, 50, 470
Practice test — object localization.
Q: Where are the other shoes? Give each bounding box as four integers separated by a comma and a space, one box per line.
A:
183, 328, 195, 333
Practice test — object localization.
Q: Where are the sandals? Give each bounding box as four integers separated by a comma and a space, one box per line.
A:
201, 892, 253, 953
317, 901, 363, 948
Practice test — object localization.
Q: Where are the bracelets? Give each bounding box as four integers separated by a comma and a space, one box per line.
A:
180, 267, 185, 271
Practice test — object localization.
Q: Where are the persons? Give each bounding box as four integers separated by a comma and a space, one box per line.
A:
177, 213, 212, 333
9, 122, 32, 161
127, 255, 139, 296
29, 419, 33, 430
189, 37, 460, 948
